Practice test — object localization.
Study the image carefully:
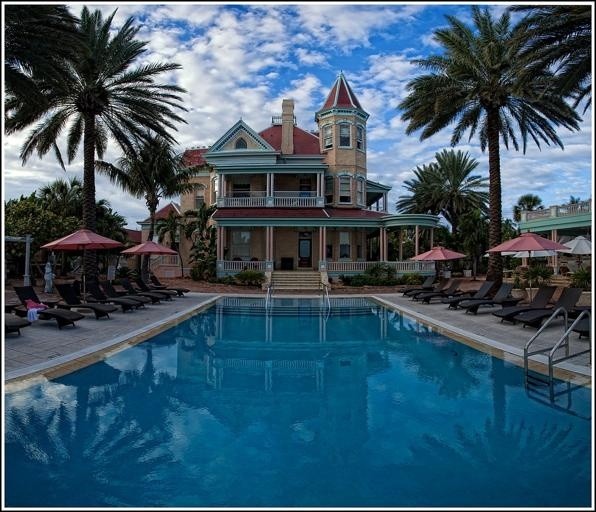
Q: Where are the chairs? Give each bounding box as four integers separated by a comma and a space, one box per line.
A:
232, 256, 258, 270
397, 274, 585, 338
12, 274, 190, 330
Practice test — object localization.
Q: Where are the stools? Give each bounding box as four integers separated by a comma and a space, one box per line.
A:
5, 311, 30, 337
567, 318, 590, 340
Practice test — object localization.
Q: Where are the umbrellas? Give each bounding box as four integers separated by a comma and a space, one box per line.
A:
407, 244, 467, 288
40, 226, 124, 302
482, 234, 592, 279
119, 238, 179, 288
484, 228, 573, 302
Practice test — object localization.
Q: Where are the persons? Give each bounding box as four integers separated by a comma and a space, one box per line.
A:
43, 261, 57, 293
